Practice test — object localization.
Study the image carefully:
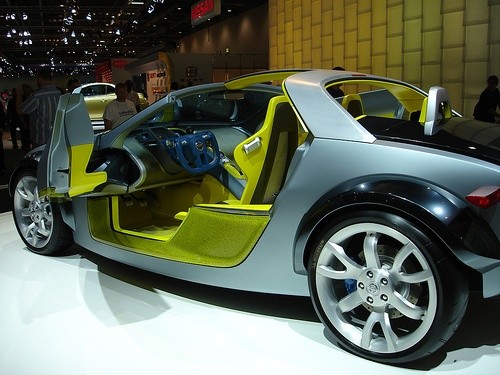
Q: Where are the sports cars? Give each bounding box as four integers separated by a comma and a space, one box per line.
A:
8, 67, 500, 366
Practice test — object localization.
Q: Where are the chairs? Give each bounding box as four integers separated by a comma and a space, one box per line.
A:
342, 95, 363, 117
174, 96, 298, 221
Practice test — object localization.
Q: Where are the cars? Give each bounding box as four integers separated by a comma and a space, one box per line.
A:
71, 82, 147, 122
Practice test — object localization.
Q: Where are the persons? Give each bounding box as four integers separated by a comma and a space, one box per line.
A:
0, 68, 80, 170
473, 76, 500, 123
325, 67, 345, 98
127, 86, 140, 113
102, 83, 138, 132
